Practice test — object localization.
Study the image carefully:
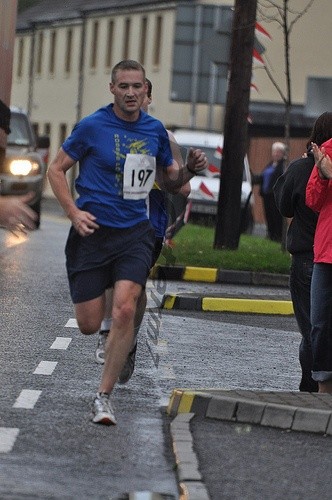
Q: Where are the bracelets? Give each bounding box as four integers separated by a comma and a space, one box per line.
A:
186, 164, 197, 175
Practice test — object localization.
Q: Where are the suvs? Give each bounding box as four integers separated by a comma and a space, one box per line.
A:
164, 124, 255, 236
0, 107, 44, 230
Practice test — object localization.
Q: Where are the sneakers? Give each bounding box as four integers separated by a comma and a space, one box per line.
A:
92, 390, 117, 425
116, 340, 138, 384
95, 329, 111, 365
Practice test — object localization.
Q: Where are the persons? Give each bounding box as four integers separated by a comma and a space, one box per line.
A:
0, 102, 38, 239
95, 76, 191, 385
273, 112, 332, 393
306, 139, 332, 393
258, 142, 288, 243
47, 60, 209, 425
